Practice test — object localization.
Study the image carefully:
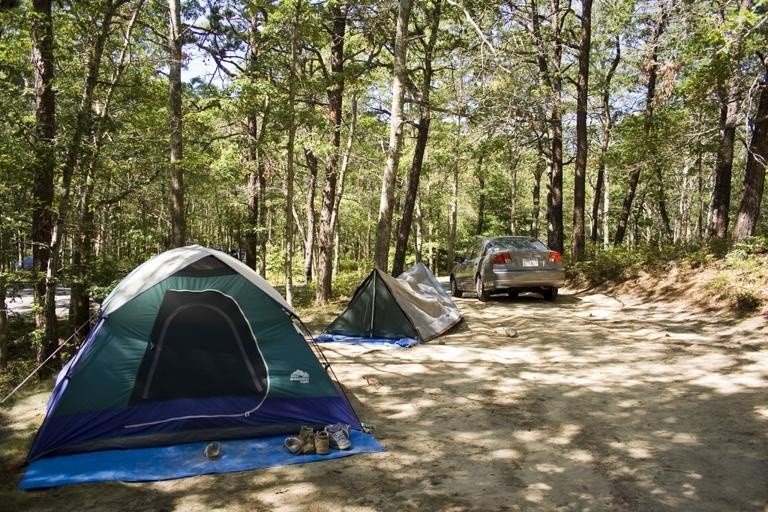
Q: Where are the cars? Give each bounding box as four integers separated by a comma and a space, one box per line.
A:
449, 234, 564, 301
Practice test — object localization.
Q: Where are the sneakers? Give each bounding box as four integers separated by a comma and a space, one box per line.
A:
284, 422, 351, 455
205, 440, 221, 458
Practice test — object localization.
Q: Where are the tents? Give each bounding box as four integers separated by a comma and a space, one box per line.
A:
18, 244, 387, 492
312, 261, 464, 349
